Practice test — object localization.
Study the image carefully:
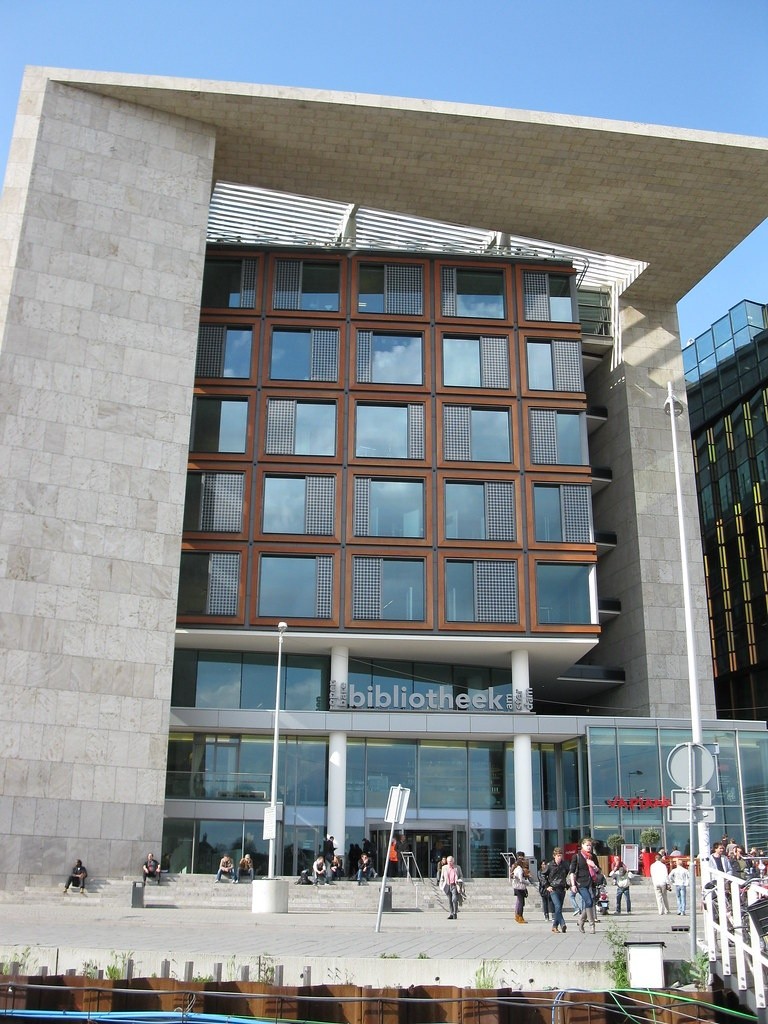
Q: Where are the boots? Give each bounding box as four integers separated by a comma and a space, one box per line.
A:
585, 907, 595, 934
577, 909, 589, 933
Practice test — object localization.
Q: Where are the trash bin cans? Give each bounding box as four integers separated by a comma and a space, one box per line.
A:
378, 885, 392, 909
524, 857, 537, 881
131, 881, 146, 908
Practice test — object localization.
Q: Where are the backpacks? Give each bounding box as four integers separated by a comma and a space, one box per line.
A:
294, 873, 312, 885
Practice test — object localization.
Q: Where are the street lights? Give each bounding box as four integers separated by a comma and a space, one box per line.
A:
663, 379, 713, 905
260, 621, 288, 879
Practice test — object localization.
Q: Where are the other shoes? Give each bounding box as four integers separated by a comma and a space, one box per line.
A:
551, 916, 560, 922
573, 909, 580, 916
660, 912, 671, 915
544, 913, 549, 921
80, 888, 84, 893
677, 911, 686, 916
453, 913, 457, 919
562, 925, 566, 933
436, 882, 439, 886
516, 915, 527, 923
63, 888, 67, 892
448, 915, 453, 919
595, 918, 599, 922
552, 928, 560, 933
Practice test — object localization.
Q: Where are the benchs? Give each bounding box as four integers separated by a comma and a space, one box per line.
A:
665, 855, 688, 872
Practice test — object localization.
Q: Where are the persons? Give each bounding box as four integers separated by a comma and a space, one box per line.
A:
312, 836, 379, 887
510, 852, 533, 923
569, 838, 602, 934
236, 854, 255, 884
541, 847, 570, 933
569, 890, 585, 917
394, 835, 411, 877
388, 838, 398, 877
62, 859, 87, 893
596, 833, 768, 916
436, 855, 447, 886
430, 842, 443, 878
583, 898, 601, 923
537, 860, 555, 922
142, 853, 161, 886
214, 854, 237, 883
439, 856, 463, 919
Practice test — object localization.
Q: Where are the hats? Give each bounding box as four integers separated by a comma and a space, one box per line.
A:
619, 867, 624, 876
245, 854, 250, 859
640, 849, 647, 853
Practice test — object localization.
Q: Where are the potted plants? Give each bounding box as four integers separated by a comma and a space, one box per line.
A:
639, 826, 661, 876
607, 833, 625, 873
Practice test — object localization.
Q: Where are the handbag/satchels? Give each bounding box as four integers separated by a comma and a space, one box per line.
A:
566, 854, 579, 886
511, 878, 526, 890
